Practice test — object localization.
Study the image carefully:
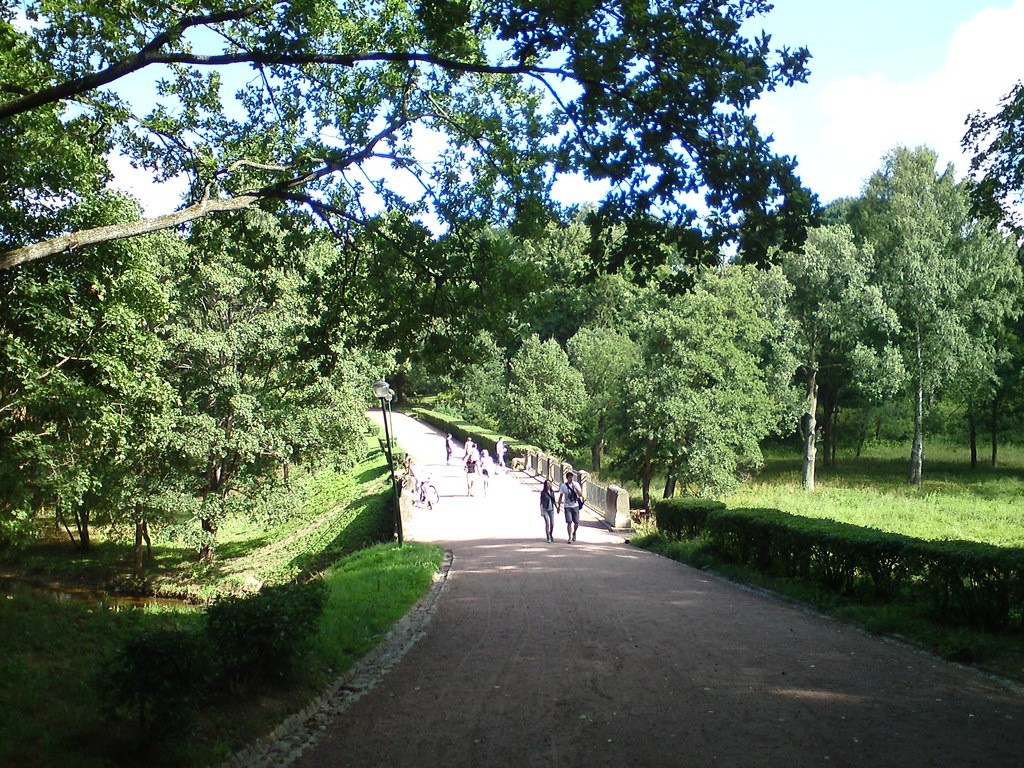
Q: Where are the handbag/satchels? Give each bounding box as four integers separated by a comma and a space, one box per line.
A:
578, 498, 582, 510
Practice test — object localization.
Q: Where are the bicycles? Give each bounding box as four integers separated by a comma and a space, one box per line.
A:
416, 476, 440, 510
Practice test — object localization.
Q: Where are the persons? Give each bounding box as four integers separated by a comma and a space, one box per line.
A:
446, 433, 507, 497
540, 478, 560, 543
557, 472, 582, 543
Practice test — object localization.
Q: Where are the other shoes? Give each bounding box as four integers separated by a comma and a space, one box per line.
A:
573, 533, 576, 540
567, 539, 571, 544
547, 536, 551, 543
551, 536, 554, 542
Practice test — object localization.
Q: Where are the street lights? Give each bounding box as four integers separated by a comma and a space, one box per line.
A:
384, 393, 394, 448
371, 379, 403, 549
